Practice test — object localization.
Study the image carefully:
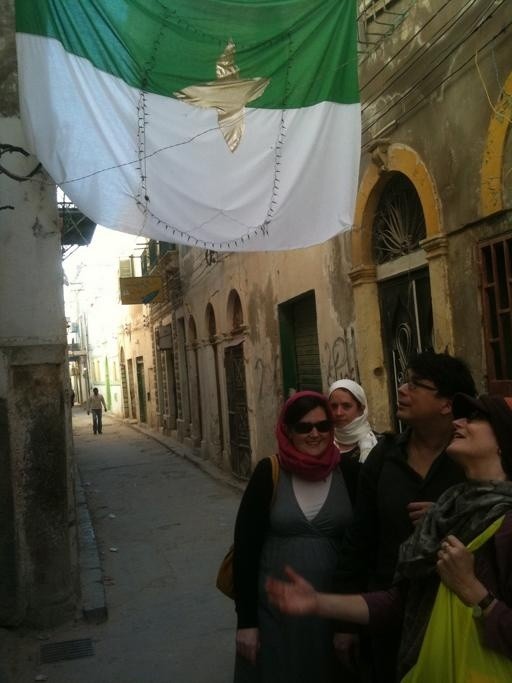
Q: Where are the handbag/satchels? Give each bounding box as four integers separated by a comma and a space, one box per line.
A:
402, 579, 512, 683
216, 546, 240, 600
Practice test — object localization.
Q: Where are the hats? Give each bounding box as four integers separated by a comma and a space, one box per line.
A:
451, 393, 512, 472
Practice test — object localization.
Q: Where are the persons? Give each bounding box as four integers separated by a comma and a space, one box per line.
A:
88, 388, 107, 434
71, 390, 75, 406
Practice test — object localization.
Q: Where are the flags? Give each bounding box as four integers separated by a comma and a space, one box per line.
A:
14, 1, 364, 254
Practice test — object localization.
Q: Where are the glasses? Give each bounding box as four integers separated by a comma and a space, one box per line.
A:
399, 378, 439, 390
295, 420, 331, 433
